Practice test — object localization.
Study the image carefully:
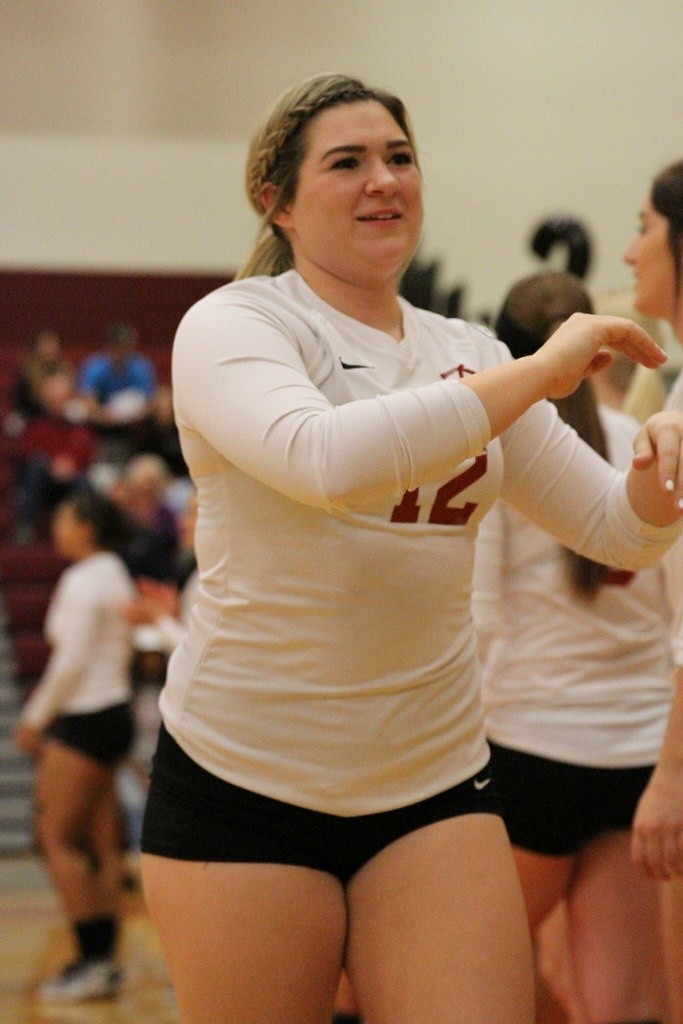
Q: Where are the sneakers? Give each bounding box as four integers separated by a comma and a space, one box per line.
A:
35, 952, 125, 1008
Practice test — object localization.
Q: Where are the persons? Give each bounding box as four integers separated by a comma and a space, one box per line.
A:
16, 494, 138, 1006
139, 74, 683, 1024
624, 160, 683, 881
8, 325, 196, 886
473, 268, 671, 1024
